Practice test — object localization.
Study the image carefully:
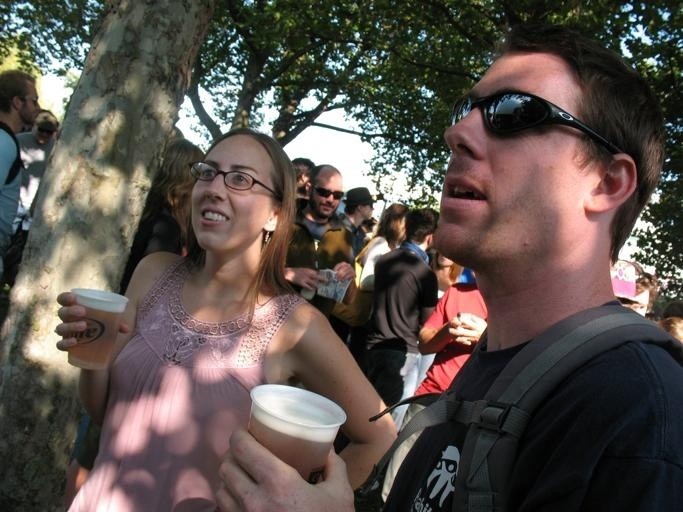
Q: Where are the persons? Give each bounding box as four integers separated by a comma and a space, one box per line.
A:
55, 129, 397, 512
212, 19, 683, 512
1, 70, 58, 292
120, 139, 205, 295
283, 158, 487, 507
657, 301, 683, 344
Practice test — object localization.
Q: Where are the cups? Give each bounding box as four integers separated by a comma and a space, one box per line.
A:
246, 382, 346, 481
66, 287, 128, 368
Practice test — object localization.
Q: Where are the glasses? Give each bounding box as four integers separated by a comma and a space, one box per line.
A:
312, 185, 345, 200
188, 160, 277, 198
450, 88, 623, 155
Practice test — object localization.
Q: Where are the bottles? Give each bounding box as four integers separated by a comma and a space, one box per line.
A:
302, 269, 320, 301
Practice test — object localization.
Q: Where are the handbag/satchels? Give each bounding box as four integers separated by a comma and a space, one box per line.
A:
331, 261, 373, 327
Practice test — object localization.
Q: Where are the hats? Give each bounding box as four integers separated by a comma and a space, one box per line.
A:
343, 188, 375, 205
